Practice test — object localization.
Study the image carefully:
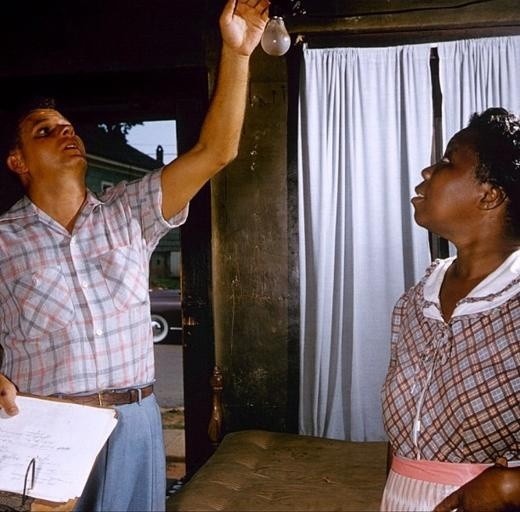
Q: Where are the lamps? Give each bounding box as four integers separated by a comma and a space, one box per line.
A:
257, 0, 294, 59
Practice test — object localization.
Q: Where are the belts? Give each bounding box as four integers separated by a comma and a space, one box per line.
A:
49, 377, 155, 410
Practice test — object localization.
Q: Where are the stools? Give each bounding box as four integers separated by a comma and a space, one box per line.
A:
162, 428, 394, 512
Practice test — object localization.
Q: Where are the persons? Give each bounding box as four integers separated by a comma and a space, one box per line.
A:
1, 0, 274, 511
379, 107, 520, 511
